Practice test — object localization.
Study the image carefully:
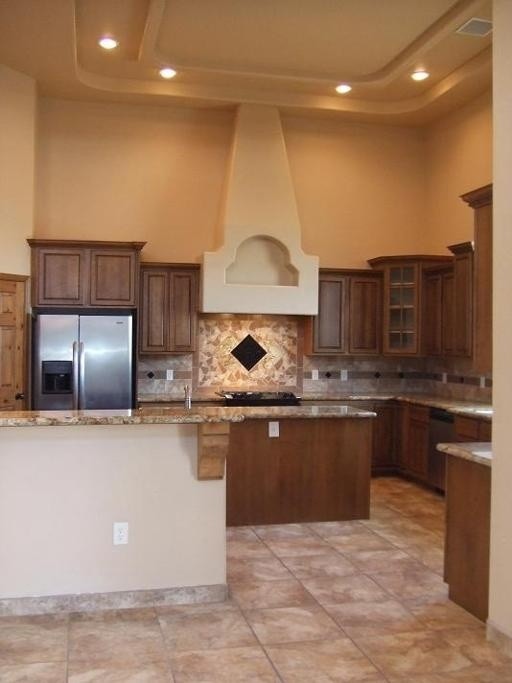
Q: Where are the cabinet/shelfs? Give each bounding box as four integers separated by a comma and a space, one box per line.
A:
366, 255, 453, 360
303, 267, 381, 358
434, 439, 491, 622
1, 271, 35, 412
27, 238, 149, 308
138, 261, 202, 356
446, 241, 475, 361
370, 399, 492, 493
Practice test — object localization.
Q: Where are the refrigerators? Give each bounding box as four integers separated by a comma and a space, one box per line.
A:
31, 312, 133, 410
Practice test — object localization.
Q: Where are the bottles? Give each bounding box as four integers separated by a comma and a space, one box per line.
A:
183, 384, 192, 409
